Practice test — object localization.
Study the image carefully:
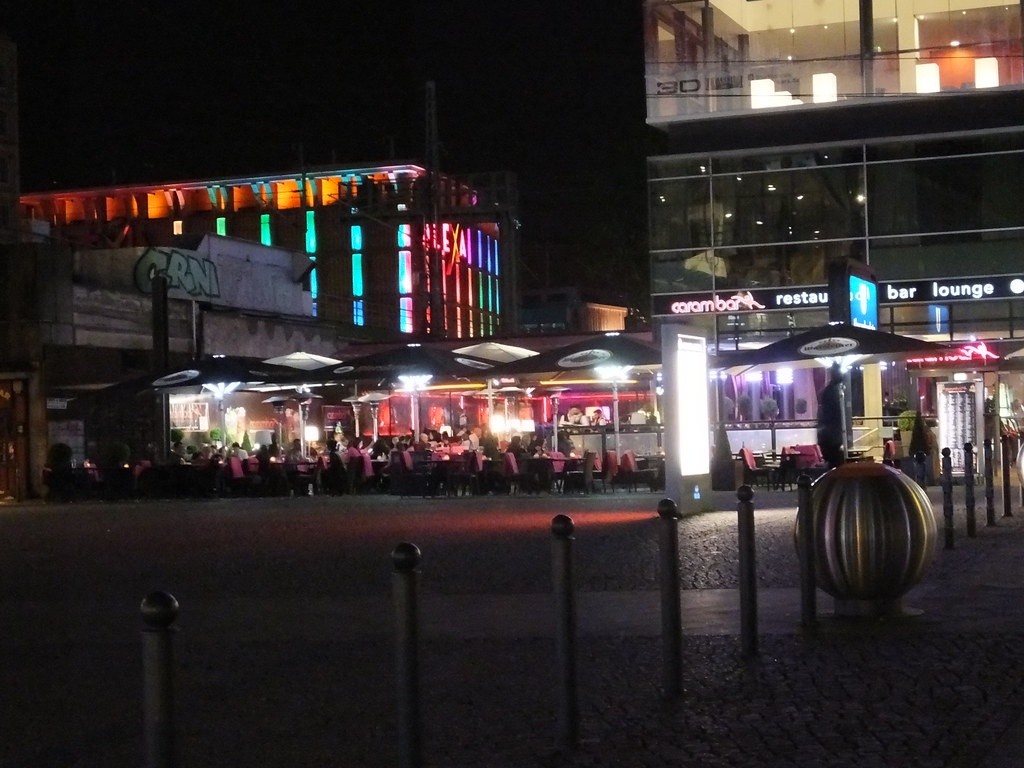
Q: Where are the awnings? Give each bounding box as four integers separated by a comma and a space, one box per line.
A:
65, 318, 875, 410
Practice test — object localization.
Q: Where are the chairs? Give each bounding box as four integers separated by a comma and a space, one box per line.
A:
740, 447, 768, 487
873, 440, 897, 463
795, 446, 820, 480
773, 448, 794, 491
41, 442, 665, 494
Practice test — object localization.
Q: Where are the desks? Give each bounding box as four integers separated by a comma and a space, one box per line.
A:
271, 462, 330, 492
370, 460, 389, 488
418, 460, 467, 497
848, 445, 874, 456
483, 459, 505, 467
547, 457, 587, 493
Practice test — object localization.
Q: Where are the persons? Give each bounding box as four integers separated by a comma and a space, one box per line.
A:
84, 458, 99, 481
168, 432, 318, 498
477, 425, 575, 497
558, 408, 610, 425
320, 420, 480, 498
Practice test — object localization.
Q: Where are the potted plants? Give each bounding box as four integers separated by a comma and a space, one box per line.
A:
171, 429, 184, 450
761, 396, 780, 420
897, 410, 918, 457
